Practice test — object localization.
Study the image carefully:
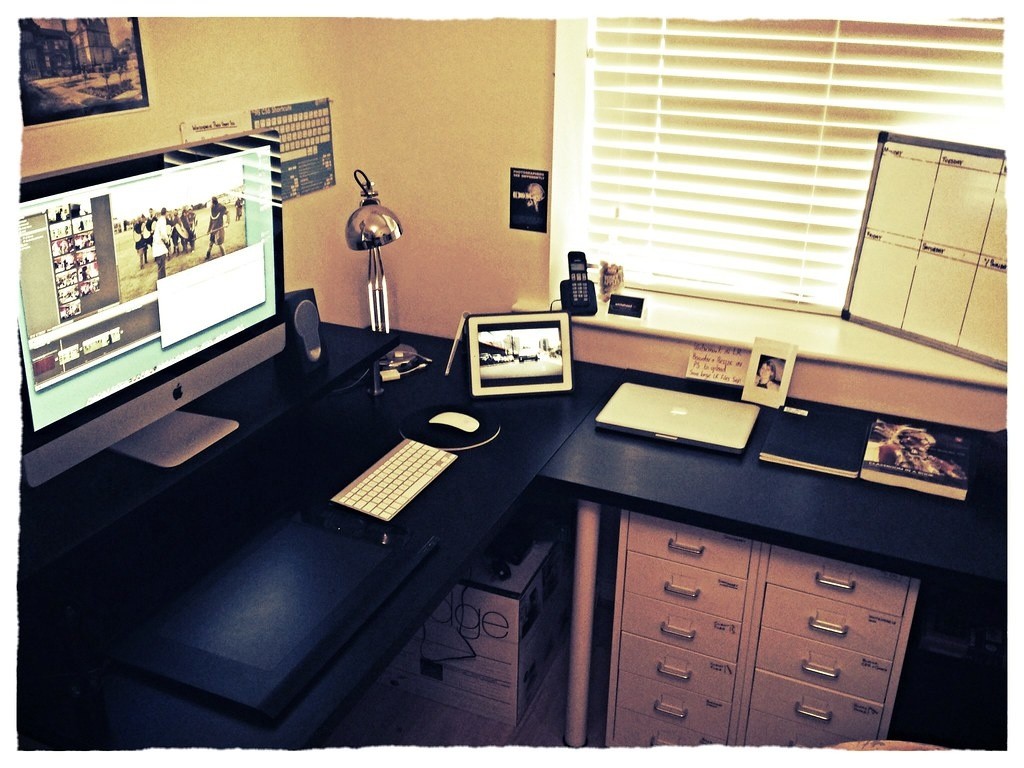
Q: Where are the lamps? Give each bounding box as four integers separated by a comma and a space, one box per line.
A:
344, 169, 433, 381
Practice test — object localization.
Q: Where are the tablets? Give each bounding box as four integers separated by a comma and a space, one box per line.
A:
466, 313, 572, 396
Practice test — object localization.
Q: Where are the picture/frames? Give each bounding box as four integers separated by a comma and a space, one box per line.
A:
465, 309, 574, 397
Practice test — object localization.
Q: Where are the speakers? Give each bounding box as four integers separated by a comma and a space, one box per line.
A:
284, 288, 329, 374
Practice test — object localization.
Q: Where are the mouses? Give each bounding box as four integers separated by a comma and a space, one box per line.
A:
429, 411, 480, 433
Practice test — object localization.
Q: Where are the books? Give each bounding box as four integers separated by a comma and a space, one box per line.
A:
860, 419, 970, 501
758, 405, 873, 478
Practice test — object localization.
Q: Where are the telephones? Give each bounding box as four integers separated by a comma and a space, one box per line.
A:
559, 250, 598, 316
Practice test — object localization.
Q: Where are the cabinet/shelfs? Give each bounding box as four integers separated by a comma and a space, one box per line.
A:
605, 505, 921, 748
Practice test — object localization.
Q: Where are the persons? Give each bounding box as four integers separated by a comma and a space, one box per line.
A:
205, 196, 230, 261
134, 205, 199, 269
756, 361, 780, 391
153, 208, 171, 290
235, 191, 245, 221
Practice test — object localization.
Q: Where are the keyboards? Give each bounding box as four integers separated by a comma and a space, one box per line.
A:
330, 439, 458, 521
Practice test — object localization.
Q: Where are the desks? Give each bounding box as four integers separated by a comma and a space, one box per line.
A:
17, 323, 622, 750
538, 368, 1007, 750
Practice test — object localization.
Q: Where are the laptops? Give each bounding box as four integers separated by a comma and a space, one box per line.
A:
595, 382, 761, 455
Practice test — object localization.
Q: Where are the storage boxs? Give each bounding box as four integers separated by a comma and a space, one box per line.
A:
373, 516, 576, 726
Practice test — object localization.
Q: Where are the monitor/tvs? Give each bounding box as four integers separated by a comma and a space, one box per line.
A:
19, 129, 286, 490
